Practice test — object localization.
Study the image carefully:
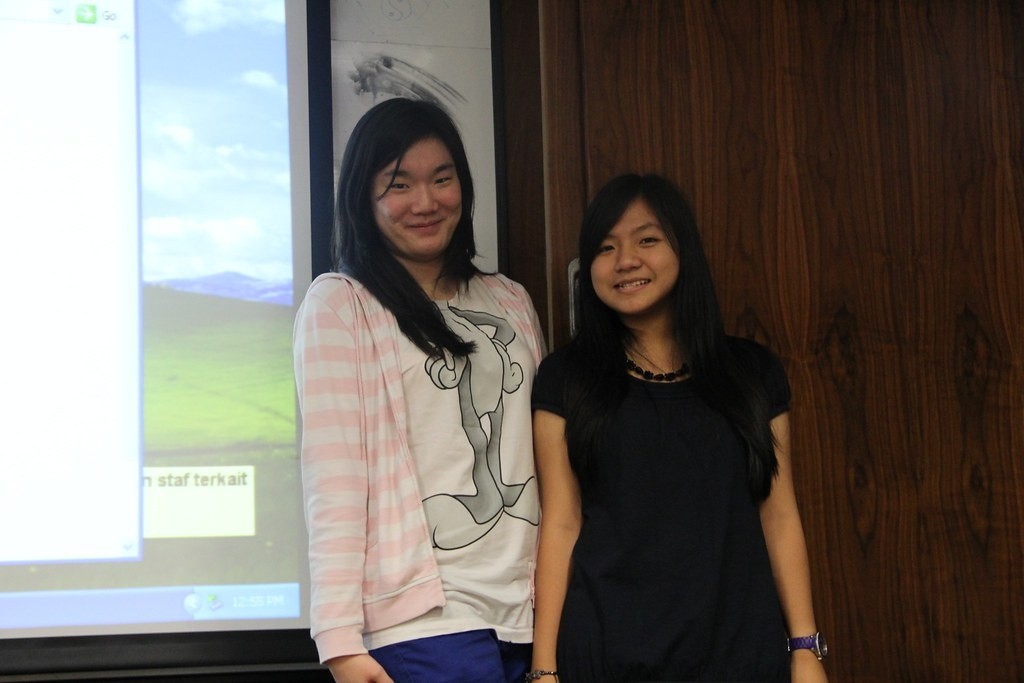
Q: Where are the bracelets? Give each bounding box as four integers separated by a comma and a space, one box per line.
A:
525, 670, 557, 683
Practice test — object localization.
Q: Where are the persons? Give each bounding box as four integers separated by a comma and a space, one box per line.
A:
296, 97, 549, 683
524, 172, 829, 683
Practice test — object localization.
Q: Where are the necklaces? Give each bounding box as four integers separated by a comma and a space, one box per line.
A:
625, 354, 687, 381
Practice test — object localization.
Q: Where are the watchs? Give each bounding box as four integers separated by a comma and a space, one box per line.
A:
786, 632, 828, 661
